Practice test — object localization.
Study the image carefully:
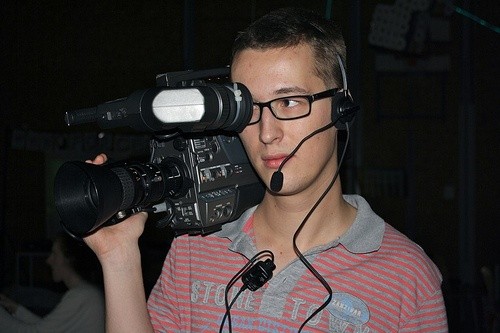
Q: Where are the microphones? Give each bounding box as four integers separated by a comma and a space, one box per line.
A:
270, 111, 349, 191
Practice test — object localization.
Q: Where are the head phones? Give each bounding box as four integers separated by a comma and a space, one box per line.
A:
308, 19, 359, 131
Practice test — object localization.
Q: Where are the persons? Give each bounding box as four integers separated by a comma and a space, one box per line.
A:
0, 232, 105, 333
82, 7, 448, 333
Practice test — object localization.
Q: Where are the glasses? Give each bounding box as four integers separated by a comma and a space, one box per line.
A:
247, 87, 339, 126
